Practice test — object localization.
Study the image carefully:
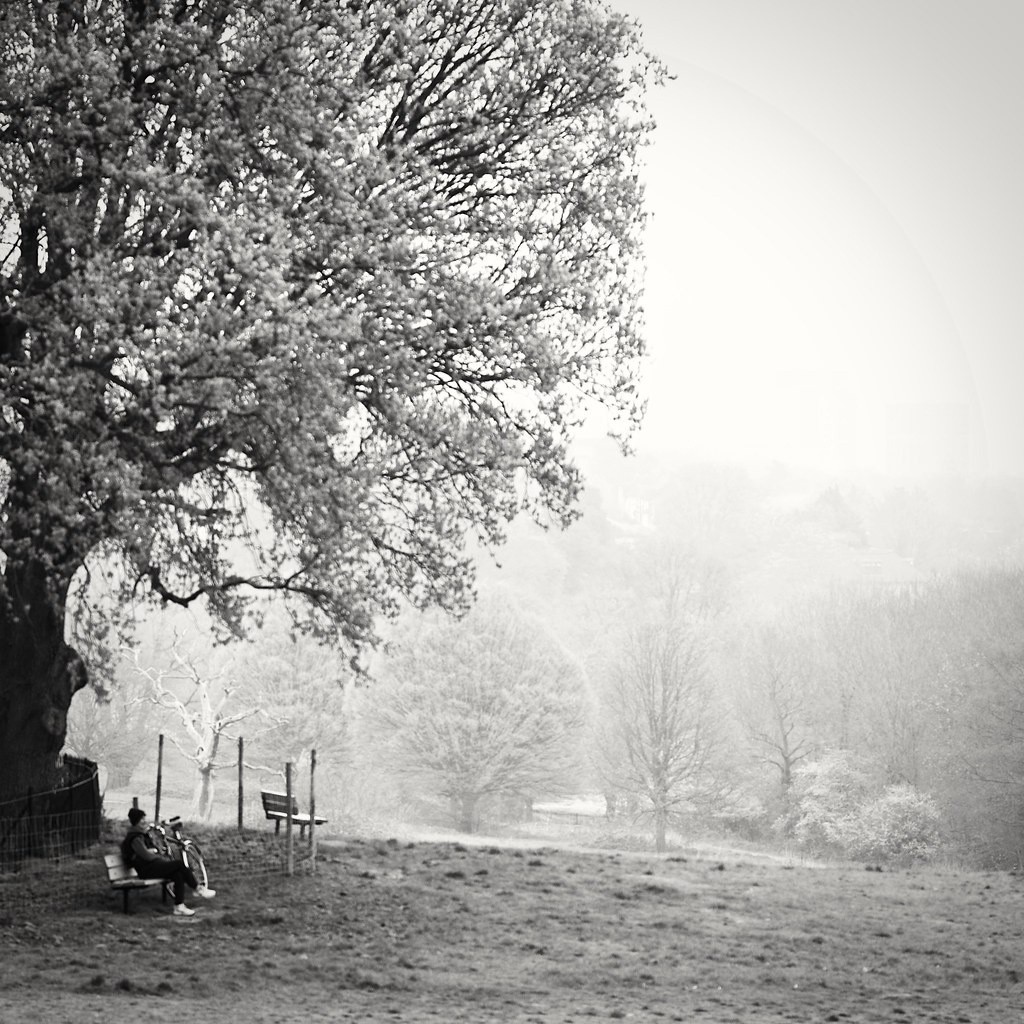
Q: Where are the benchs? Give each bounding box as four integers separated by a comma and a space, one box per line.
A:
259, 789, 327, 839
104, 848, 177, 911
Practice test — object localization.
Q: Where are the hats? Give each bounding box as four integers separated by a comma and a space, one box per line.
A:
127, 808, 145, 826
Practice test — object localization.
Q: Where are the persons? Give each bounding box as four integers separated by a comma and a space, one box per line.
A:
122, 808, 216, 917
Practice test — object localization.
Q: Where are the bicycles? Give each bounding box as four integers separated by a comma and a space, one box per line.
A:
146, 815, 209, 898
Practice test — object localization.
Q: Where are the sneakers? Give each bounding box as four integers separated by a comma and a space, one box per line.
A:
193, 884, 216, 898
173, 902, 196, 916
